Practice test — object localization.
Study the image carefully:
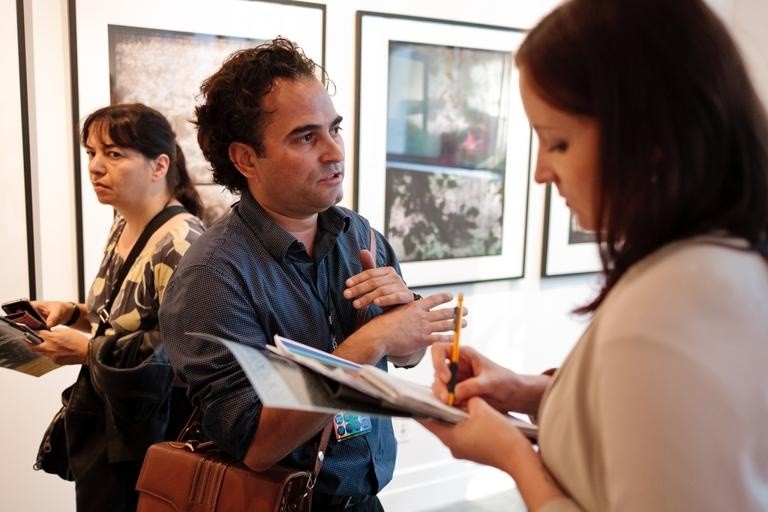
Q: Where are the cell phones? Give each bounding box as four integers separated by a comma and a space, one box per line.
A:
2, 300, 48, 328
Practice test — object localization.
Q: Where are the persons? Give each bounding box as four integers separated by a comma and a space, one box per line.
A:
23, 103, 208, 512
135, 37, 468, 512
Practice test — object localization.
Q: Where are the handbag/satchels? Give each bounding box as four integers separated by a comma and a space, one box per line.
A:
134, 440, 315, 511
31, 383, 103, 483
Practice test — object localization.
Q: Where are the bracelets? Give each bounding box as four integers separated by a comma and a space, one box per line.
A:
64, 303, 80, 326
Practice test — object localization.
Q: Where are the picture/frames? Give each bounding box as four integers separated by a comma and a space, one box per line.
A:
68, 0, 327, 305
353, 11, 534, 290
541, 177, 616, 278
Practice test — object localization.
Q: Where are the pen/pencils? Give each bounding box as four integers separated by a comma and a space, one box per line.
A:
448, 292, 463, 408
24, 332, 41, 344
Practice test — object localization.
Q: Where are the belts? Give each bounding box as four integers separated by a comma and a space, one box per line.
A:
315, 492, 371, 509
